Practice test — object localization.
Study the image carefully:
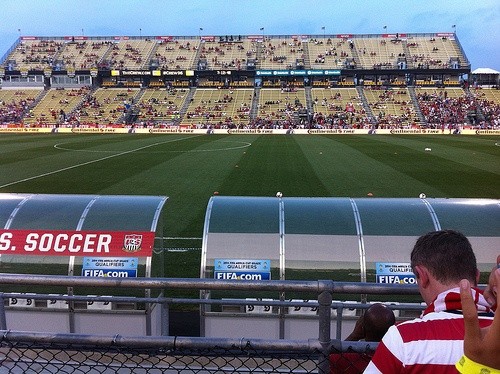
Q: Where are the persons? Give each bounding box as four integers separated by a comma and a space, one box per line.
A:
0, 35, 500, 129
331, 230, 500, 374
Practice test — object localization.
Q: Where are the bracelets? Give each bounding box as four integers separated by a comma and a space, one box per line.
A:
455, 354, 500, 373
